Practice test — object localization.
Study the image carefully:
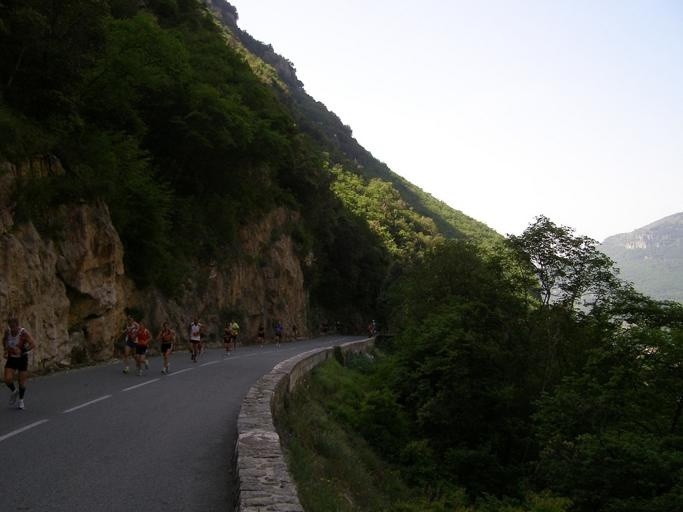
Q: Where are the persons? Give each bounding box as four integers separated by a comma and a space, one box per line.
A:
257, 321, 264, 352
230, 319, 240, 349
119, 315, 140, 375
1, 317, 35, 410
272, 319, 283, 352
223, 322, 233, 356
198, 323, 207, 354
156, 321, 175, 374
129, 320, 152, 376
187, 317, 201, 362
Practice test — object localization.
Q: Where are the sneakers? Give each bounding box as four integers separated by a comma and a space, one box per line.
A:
17, 398, 25, 409
144, 360, 149, 369
134, 366, 142, 376
160, 366, 169, 375
122, 366, 130, 376
8, 388, 20, 405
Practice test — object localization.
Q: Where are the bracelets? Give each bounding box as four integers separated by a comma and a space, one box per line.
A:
21, 347, 27, 353
